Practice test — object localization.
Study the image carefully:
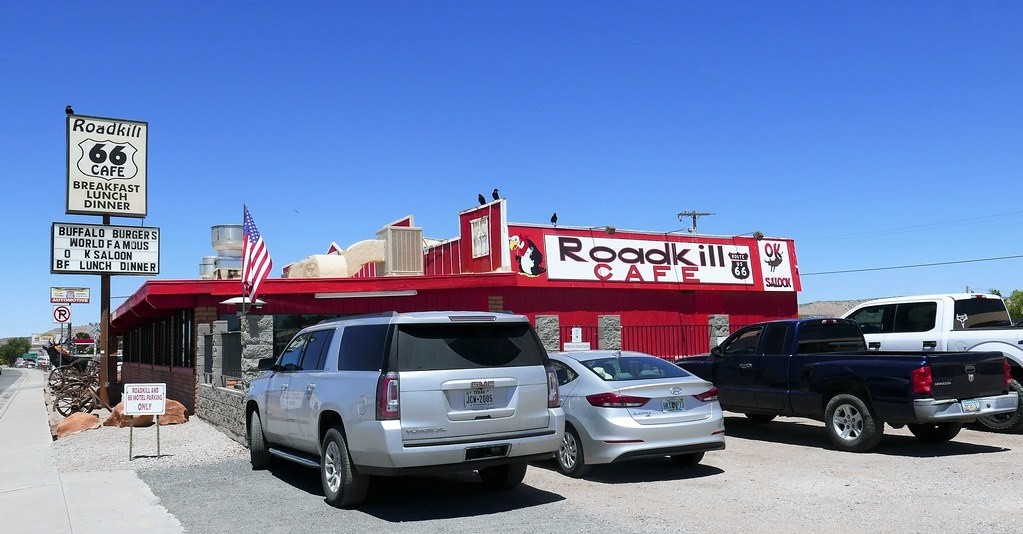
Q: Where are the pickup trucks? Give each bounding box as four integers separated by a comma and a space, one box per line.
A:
677, 316, 1018, 453
839, 293, 1023, 433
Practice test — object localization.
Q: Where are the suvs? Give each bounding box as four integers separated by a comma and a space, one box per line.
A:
245, 310, 564, 506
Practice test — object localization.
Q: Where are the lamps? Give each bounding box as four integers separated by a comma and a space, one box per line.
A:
606, 226, 615, 233
754, 231, 765, 241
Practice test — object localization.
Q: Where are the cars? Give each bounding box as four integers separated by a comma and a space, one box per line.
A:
546, 351, 727, 479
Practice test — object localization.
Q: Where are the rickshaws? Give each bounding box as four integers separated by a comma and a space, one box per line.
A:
43, 339, 100, 392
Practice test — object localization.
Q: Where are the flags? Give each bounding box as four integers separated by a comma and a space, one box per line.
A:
240, 203, 272, 303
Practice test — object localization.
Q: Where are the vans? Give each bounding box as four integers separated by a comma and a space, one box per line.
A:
37, 356, 49, 367
